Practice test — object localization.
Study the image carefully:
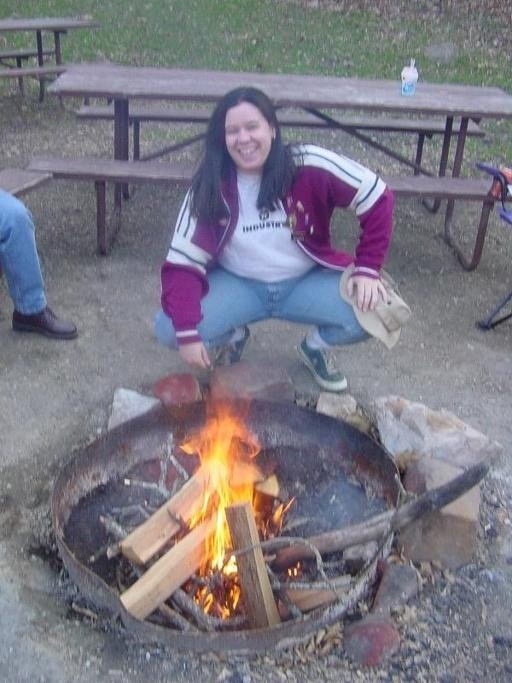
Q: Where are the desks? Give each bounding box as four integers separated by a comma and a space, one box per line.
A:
47, 54, 512, 215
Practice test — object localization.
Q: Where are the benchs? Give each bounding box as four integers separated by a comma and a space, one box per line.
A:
65, 103, 485, 212
0, 6, 105, 202
24, 146, 512, 275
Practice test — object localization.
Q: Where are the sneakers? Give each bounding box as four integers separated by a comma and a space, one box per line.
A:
296, 337, 349, 391
214, 325, 252, 367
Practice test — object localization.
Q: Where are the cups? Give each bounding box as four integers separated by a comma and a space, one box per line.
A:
399, 65, 419, 96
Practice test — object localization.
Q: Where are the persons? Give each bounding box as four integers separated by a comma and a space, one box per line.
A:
0, 189, 78, 338
156, 87, 403, 391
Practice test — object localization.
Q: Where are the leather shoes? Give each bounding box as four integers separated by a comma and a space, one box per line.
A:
13, 308, 79, 340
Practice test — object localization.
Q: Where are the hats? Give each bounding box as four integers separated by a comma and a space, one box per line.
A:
340, 262, 412, 352
